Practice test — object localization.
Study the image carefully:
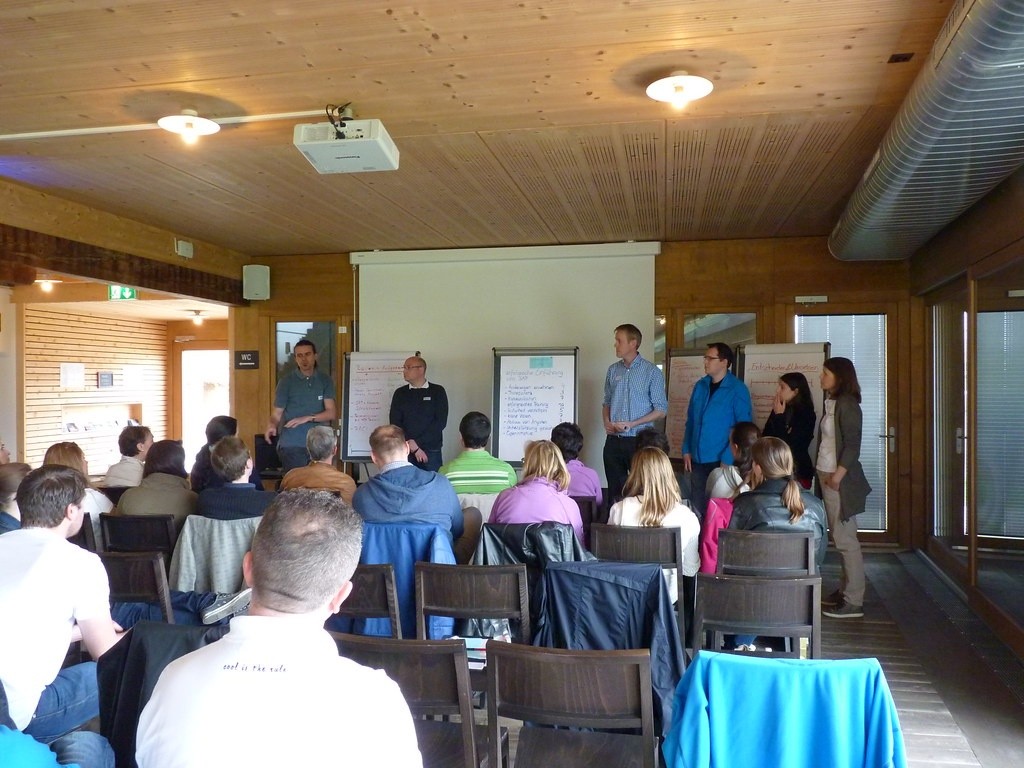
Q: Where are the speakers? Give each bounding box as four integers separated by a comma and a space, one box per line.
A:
243, 265, 270, 300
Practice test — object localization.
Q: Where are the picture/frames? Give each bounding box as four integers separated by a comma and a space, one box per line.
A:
97, 371, 115, 389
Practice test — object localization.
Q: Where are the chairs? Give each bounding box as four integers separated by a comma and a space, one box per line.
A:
486, 639, 660, 768
325, 629, 510, 768
94, 622, 232, 768
66, 470, 823, 721
663, 648, 908, 768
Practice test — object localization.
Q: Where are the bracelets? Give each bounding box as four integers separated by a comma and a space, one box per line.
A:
312, 414, 315, 422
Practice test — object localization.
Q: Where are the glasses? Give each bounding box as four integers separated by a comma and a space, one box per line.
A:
703, 355, 724, 363
400, 365, 423, 371
521, 458, 525, 464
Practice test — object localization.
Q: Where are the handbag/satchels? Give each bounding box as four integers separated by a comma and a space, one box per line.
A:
442, 630, 512, 710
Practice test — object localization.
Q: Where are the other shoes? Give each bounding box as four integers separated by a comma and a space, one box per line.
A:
735, 644, 774, 653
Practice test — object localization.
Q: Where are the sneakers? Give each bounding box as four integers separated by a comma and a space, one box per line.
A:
200, 587, 252, 624
822, 598, 864, 617
822, 589, 845, 605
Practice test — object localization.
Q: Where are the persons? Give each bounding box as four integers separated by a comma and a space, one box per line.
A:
135, 487, 424, 768
761, 372, 817, 490
682, 342, 753, 516
264, 340, 337, 448
0, 464, 134, 768
276, 411, 827, 640
814, 357, 872, 618
389, 357, 449, 472
603, 324, 668, 507
0, 416, 279, 627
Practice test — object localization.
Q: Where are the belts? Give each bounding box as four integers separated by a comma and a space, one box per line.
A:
607, 434, 638, 439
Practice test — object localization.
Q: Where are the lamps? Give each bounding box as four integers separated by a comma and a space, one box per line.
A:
156, 108, 222, 145
33, 275, 63, 292
644, 69, 714, 112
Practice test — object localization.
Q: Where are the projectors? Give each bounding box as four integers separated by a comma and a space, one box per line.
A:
293, 119, 399, 175
